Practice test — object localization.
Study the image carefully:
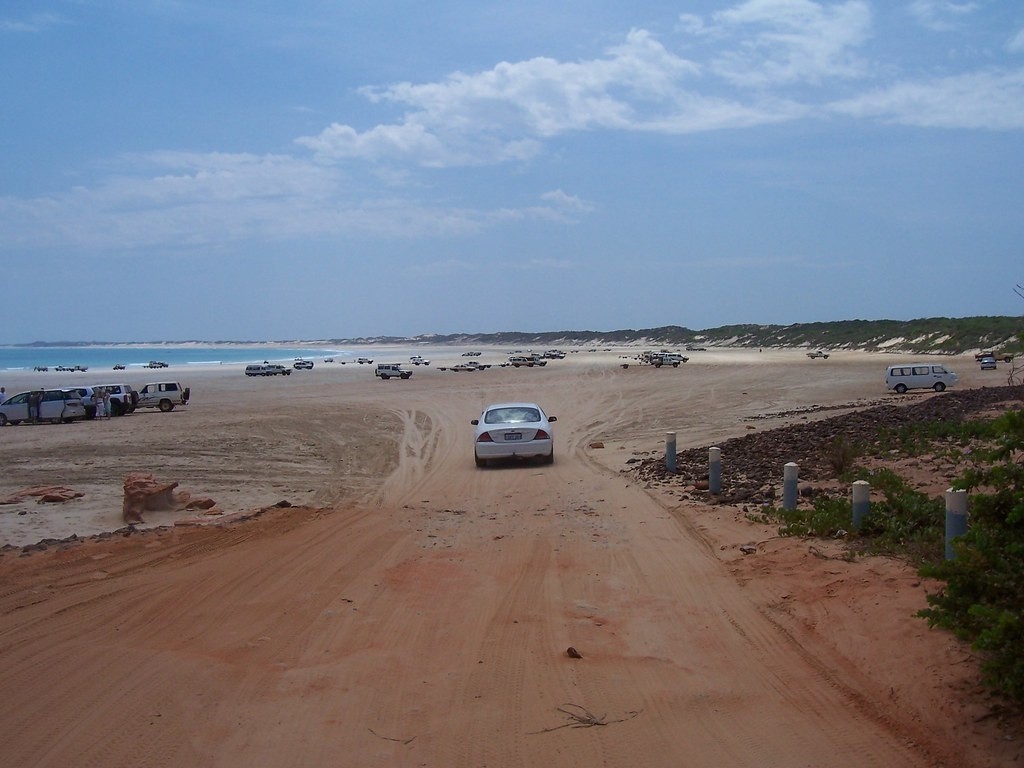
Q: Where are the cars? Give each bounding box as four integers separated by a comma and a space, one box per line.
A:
471, 403, 556, 464
450, 365, 475, 372
980, 358, 997, 369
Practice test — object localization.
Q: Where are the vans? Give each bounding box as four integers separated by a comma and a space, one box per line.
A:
0, 389, 85, 426
245, 364, 272, 376
884, 364, 959, 391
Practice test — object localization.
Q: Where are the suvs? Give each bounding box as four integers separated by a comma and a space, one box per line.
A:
412, 357, 430, 365
267, 365, 292, 376
138, 382, 190, 412
60, 387, 103, 419
294, 361, 313, 369
552, 353, 564, 359
376, 363, 413, 380
324, 357, 333, 362
512, 358, 535, 368
358, 358, 374, 364
92, 385, 139, 417
531, 353, 543, 359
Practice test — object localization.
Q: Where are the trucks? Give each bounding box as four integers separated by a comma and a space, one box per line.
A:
808, 351, 830, 360
35, 360, 169, 372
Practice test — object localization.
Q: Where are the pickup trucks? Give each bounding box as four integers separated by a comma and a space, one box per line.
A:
521, 357, 547, 367
465, 361, 485, 370
651, 357, 679, 367
651, 353, 689, 363
975, 351, 1014, 363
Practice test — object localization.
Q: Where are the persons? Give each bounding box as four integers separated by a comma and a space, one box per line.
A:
28, 392, 40, 425
0, 387, 8, 408
94, 388, 104, 421
103, 389, 112, 421
